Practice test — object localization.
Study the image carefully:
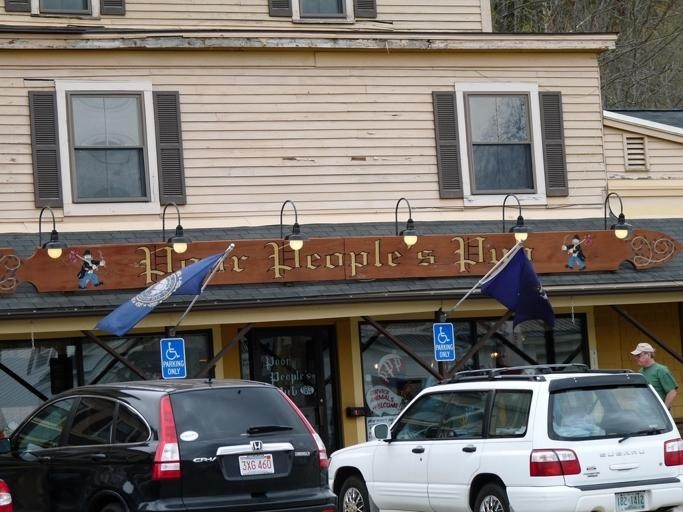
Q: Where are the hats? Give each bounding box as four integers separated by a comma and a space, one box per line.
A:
631, 343, 656, 356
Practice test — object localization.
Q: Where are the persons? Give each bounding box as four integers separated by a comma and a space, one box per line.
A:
630, 343, 679, 410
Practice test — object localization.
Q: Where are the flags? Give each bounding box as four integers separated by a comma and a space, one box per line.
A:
94, 252, 227, 339
479, 244, 555, 328
